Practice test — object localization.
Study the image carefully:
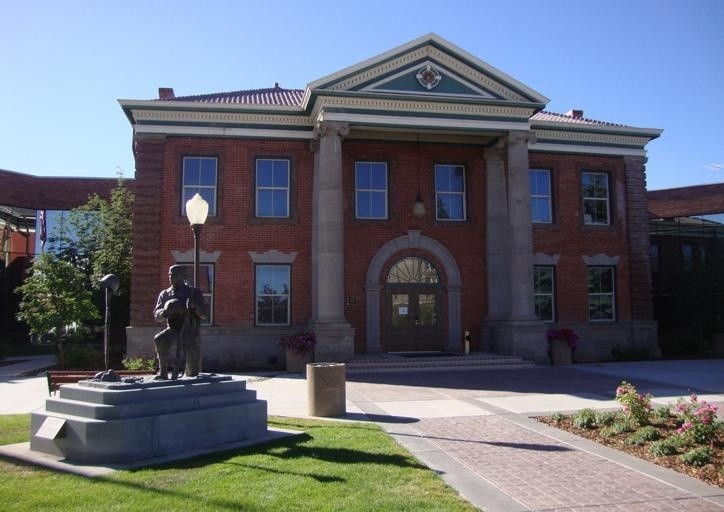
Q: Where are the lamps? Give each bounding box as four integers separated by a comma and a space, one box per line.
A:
412, 133, 426, 220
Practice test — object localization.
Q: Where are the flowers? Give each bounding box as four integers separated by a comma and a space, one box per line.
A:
276, 328, 316, 357
546, 326, 579, 348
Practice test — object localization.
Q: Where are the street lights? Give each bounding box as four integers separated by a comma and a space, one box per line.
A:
183, 191, 211, 377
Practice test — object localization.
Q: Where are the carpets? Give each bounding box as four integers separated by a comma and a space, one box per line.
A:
389, 352, 464, 358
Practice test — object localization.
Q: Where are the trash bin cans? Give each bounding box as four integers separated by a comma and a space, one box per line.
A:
307, 362, 347, 418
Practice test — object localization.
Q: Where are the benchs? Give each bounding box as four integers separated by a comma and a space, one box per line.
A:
44, 370, 158, 397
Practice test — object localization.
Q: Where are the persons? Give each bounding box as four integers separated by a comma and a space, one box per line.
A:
151, 263, 210, 379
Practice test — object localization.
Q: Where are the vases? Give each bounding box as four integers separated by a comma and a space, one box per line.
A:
285, 348, 310, 373
551, 338, 572, 365
